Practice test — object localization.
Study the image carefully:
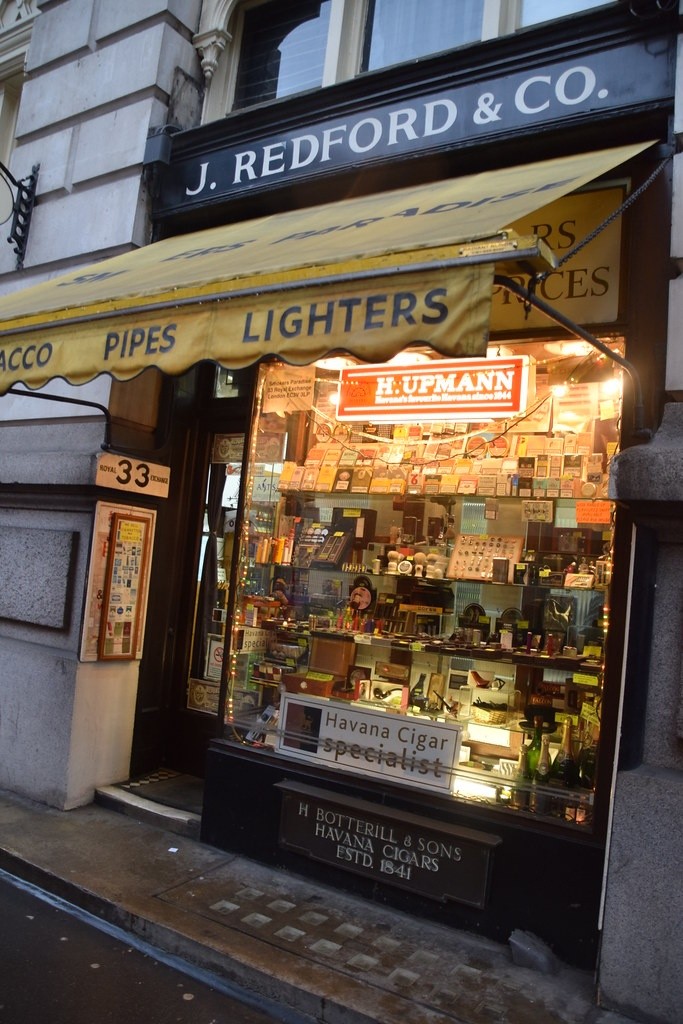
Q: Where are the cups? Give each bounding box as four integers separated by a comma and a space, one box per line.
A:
372, 559, 380, 574
576, 634, 585, 653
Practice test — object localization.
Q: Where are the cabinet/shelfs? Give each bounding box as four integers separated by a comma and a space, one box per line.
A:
231, 488, 618, 807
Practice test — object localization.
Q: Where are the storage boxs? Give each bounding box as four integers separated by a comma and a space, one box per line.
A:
277, 422, 610, 590
281, 629, 358, 697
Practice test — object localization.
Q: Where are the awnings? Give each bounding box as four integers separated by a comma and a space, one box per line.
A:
0, 141, 670, 427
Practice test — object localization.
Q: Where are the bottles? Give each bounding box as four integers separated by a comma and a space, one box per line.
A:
510, 744, 530, 809
527, 716, 551, 778
587, 792, 595, 824
575, 718, 585, 757
576, 796, 587, 824
551, 717, 578, 818
501, 624, 512, 651
531, 734, 551, 814
530, 566, 537, 585
564, 793, 575, 822
581, 753, 595, 789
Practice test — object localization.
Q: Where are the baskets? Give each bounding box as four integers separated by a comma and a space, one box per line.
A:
470, 703, 513, 725
